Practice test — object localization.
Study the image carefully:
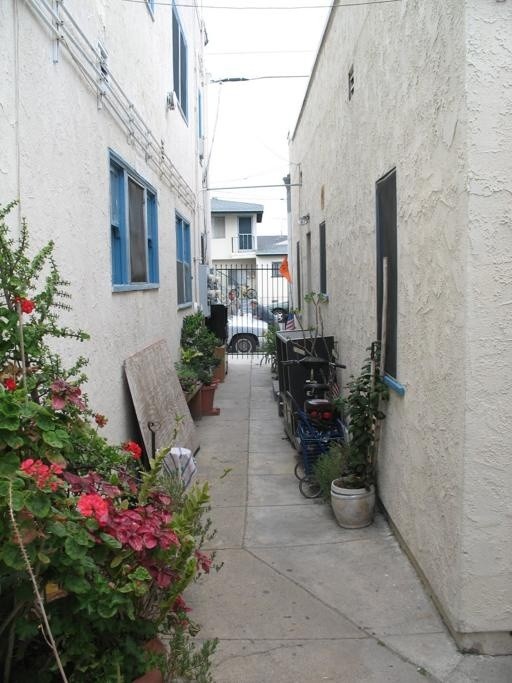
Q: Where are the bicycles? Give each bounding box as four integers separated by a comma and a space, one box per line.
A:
228, 280, 257, 301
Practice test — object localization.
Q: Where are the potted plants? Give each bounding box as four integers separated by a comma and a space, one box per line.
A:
178, 308, 222, 420
329, 341, 387, 528
260, 323, 283, 392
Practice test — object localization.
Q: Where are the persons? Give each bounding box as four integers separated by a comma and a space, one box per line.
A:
249, 298, 278, 325
225, 289, 242, 316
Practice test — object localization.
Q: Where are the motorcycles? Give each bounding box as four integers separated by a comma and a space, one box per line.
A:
274, 353, 351, 428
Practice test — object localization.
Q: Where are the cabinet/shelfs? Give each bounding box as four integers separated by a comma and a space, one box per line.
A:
275, 330, 334, 434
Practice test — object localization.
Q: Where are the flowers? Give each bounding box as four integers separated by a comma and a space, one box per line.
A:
0, 208, 223, 683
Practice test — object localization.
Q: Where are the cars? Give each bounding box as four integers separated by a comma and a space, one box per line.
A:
206, 301, 289, 354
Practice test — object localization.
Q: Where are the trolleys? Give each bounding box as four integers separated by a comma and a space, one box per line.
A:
276, 387, 349, 499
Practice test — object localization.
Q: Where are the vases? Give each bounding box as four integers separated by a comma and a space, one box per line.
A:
116, 635, 168, 681
